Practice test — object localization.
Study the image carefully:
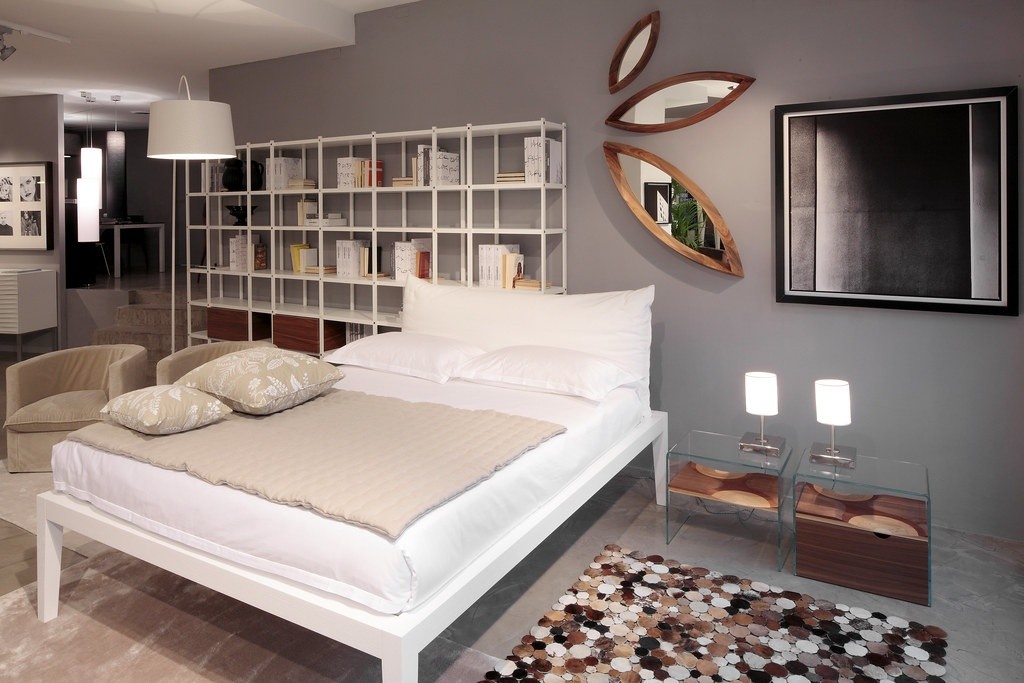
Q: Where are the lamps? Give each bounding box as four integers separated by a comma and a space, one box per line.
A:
0, 26, 16, 62
738, 370, 786, 457
74, 91, 102, 244
806, 378, 858, 470
140, 74, 240, 355
106, 95, 127, 219
77, 98, 104, 214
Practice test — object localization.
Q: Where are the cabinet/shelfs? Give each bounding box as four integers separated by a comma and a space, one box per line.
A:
184, 117, 569, 362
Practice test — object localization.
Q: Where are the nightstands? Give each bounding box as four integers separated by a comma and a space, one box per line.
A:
665, 429, 795, 573
791, 447, 933, 608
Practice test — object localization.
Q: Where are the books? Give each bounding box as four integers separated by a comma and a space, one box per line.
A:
201, 163, 228, 192
290, 238, 433, 281
266, 157, 316, 191
392, 145, 459, 187
229, 235, 267, 271
479, 244, 552, 289
337, 158, 383, 188
297, 199, 348, 226
496, 137, 561, 184
346, 322, 373, 345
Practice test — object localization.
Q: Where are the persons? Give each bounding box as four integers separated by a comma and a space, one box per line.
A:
21, 211, 38, 236
0, 209, 13, 236
20, 176, 40, 202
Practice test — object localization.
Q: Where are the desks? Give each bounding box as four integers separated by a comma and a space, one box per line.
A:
103, 223, 167, 278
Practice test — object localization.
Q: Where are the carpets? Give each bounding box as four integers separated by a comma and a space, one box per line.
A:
477, 542, 948, 683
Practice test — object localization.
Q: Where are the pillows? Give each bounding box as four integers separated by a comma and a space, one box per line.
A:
100, 384, 233, 436
173, 345, 347, 415
448, 344, 637, 404
321, 331, 487, 386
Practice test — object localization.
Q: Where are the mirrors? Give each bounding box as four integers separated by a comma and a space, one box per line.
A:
607, 9, 661, 95
604, 69, 758, 134
601, 137, 744, 279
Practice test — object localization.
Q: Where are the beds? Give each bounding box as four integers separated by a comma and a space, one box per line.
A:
34, 271, 670, 683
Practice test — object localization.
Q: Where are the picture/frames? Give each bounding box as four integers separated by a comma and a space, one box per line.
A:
643, 182, 672, 224
0, 160, 55, 252
773, 85, 1020, 317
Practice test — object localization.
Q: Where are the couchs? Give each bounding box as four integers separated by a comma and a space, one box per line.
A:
154, 340, 278, 386
2, 343, 149, 474
